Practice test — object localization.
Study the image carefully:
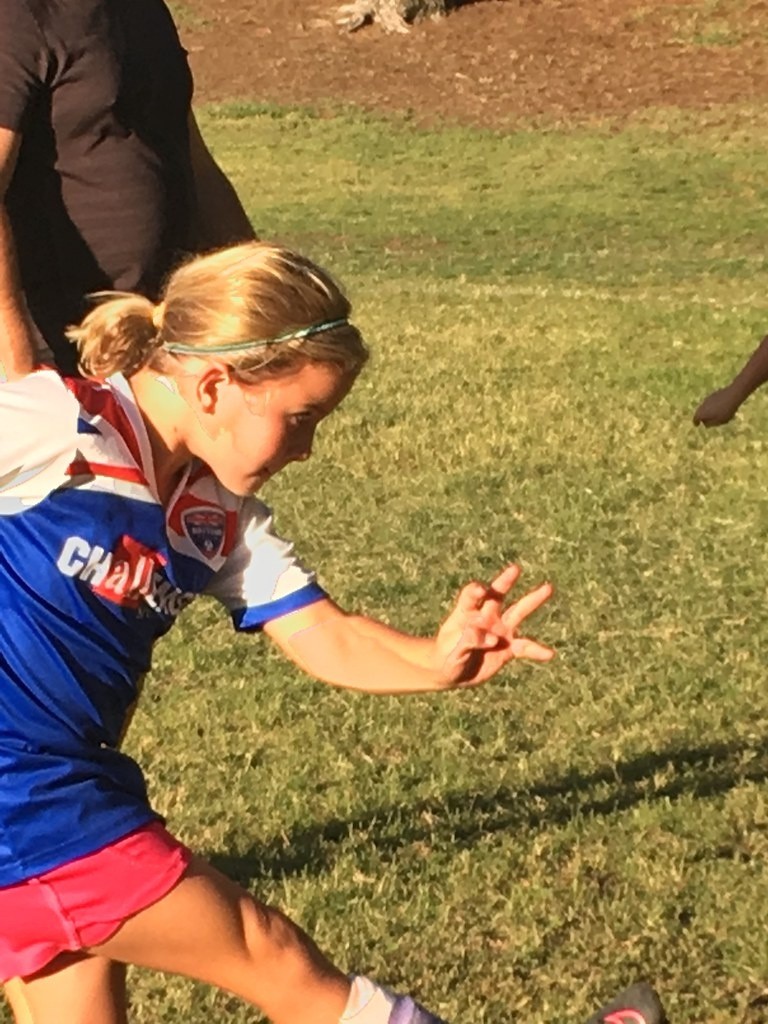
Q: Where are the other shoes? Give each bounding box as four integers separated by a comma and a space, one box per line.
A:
582, 981, 664, 1024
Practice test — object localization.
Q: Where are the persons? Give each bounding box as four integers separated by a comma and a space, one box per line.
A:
0, 0, 259, 388
0, 242, 668, 1024
